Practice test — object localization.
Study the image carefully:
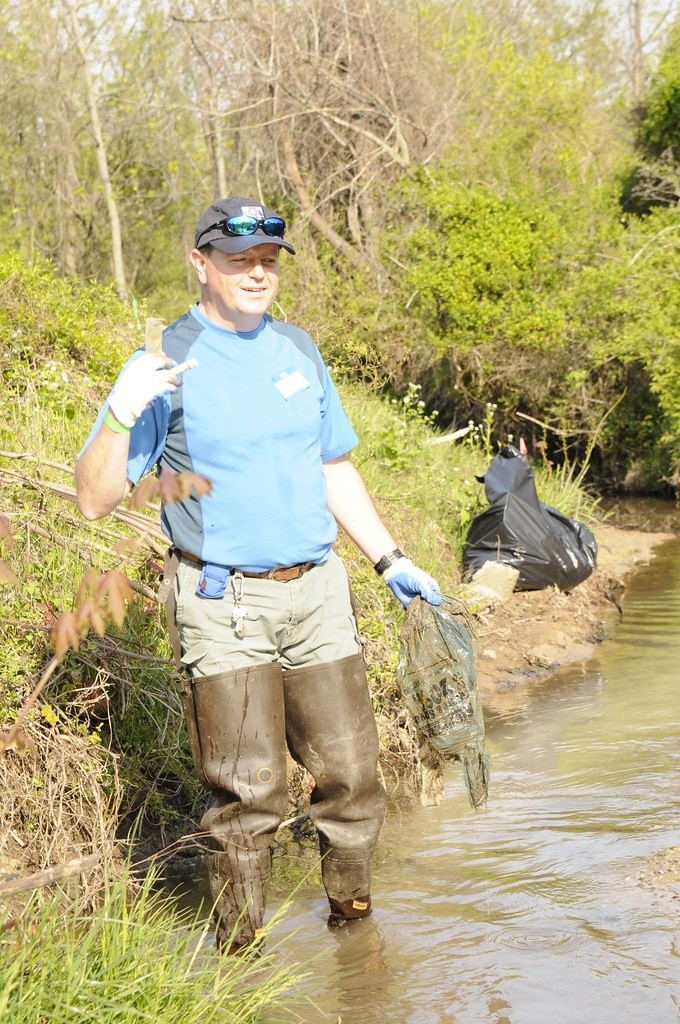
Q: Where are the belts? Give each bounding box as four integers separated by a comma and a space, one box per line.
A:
178, 549, 316, 581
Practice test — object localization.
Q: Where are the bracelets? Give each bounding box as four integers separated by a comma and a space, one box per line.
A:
102, 411, 130, 434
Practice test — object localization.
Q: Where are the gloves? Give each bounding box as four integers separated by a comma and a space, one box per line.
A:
383, 557, 443, 607
107, 348, 184, 429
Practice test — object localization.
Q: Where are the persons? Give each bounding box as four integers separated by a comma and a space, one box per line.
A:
74, 197, 442, 961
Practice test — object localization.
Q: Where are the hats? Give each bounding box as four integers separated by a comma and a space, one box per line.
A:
194, 198, 294, 256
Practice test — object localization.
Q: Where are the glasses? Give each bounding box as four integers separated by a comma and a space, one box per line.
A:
197, 214, 287, 246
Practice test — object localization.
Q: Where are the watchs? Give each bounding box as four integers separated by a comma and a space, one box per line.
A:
373, 549, 405, 576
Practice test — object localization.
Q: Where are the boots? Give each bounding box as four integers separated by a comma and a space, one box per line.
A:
285, 656, 386, 930
185, 666, 287, 955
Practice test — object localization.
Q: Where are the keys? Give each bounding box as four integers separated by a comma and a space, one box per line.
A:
231, 606, 247, 639
